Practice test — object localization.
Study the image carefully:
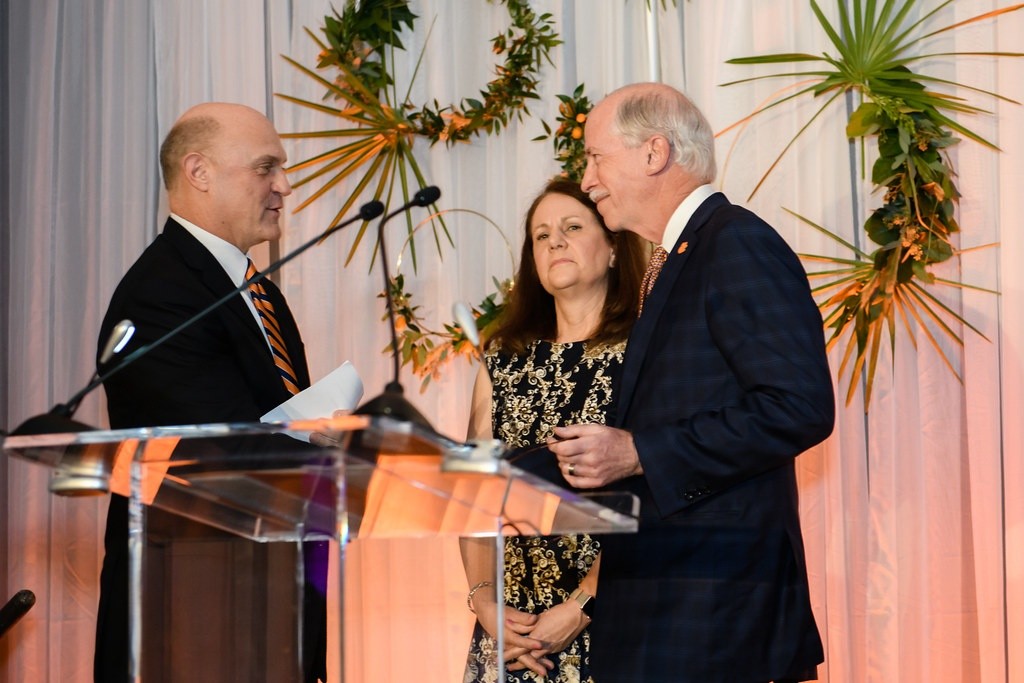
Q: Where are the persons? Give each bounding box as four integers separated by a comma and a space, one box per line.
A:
548, 82, 837, 683
459, 178, 648, 683
90, 101, 354, 683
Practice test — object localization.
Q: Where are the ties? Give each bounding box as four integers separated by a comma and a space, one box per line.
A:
636, 246, 667, 318
246, 258, 300, 397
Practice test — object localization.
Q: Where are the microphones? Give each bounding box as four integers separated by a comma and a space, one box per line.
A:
12, 200, 385, 436
349, 186, 442, 432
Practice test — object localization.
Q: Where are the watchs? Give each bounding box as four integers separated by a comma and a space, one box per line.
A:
570, 586, 596, 620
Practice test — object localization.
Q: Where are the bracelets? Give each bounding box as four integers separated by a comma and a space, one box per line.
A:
467, 580, 496, 614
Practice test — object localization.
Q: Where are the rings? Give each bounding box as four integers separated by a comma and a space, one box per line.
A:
567, 462, 577, 477
